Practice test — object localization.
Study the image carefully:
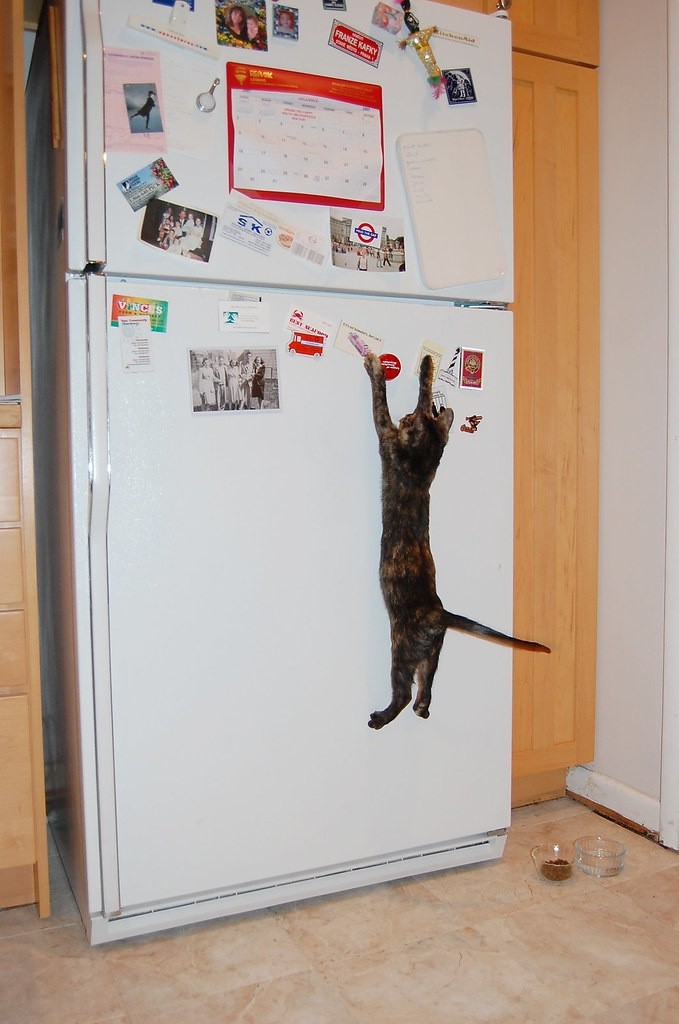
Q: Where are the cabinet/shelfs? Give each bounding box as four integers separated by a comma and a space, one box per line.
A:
0, 0, 53, 916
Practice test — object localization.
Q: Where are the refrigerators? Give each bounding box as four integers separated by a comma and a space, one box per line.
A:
26, 0, 516, 946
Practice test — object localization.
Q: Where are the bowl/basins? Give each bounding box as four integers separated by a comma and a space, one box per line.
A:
532, 845, 580, 882
576, 837, 627, 877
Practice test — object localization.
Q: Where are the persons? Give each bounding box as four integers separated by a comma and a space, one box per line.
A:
225, 6, 266, 49
131, 91, 156, 129
156, 207, 204, 256
332, 236, 405, 272
277, 11, 294, 34
445, 72, 466, 101
198, 352, 265, 411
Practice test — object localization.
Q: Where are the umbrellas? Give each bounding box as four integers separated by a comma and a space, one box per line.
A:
449, 70, 471, 85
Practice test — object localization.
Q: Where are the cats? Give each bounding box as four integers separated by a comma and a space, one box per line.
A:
363, 353, 552, 732
